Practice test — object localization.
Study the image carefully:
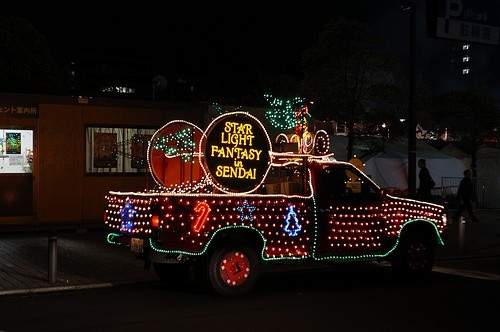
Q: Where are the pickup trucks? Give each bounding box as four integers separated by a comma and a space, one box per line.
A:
102, 153, 448, 294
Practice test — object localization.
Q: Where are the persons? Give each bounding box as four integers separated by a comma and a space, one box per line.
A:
416, 166, 435, 200
453, 169, 480, 224
